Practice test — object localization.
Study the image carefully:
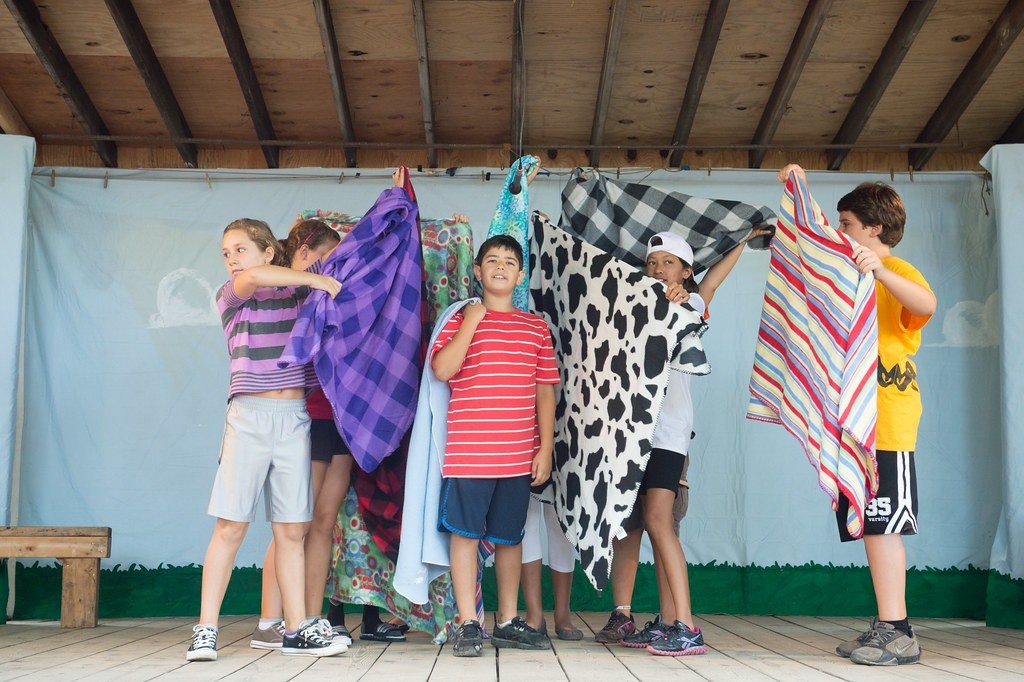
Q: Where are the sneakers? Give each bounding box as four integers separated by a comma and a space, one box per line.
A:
310, 618, 351, 646
250, 620, 285, 650
849, 622, 920, 666
835, 615, 877, 657
280, 622, 348, 656
186, 624, 218, 661
594, 610, 636, 642
646, 621, 707, 656
452, 619, 484, 656
491, 612, 551, 650
620, 616, 674, 648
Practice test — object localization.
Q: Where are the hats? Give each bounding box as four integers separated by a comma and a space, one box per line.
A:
646, 230, 694, 282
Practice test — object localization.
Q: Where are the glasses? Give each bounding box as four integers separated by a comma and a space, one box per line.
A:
304, 222, 332, 243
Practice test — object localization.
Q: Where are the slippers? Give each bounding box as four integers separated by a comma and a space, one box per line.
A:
331, 625, 353, 643
359, 623, 407, 642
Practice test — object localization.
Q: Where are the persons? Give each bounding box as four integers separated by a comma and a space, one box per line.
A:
623, 233, 708, 656
521, 155, 583, 639
328, 170, 469, 643
778, 164, 936, 666
186, 219, 347, 660
251, 221, 352, 650
430, 235, 551, 658
585, 167, 772, 645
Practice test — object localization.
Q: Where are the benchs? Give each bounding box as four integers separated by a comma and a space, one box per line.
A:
0, 525, 113, 628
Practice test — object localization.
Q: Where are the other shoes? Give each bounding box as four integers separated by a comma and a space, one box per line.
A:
554, 626, 583, 641
520, 619, 548, 640
